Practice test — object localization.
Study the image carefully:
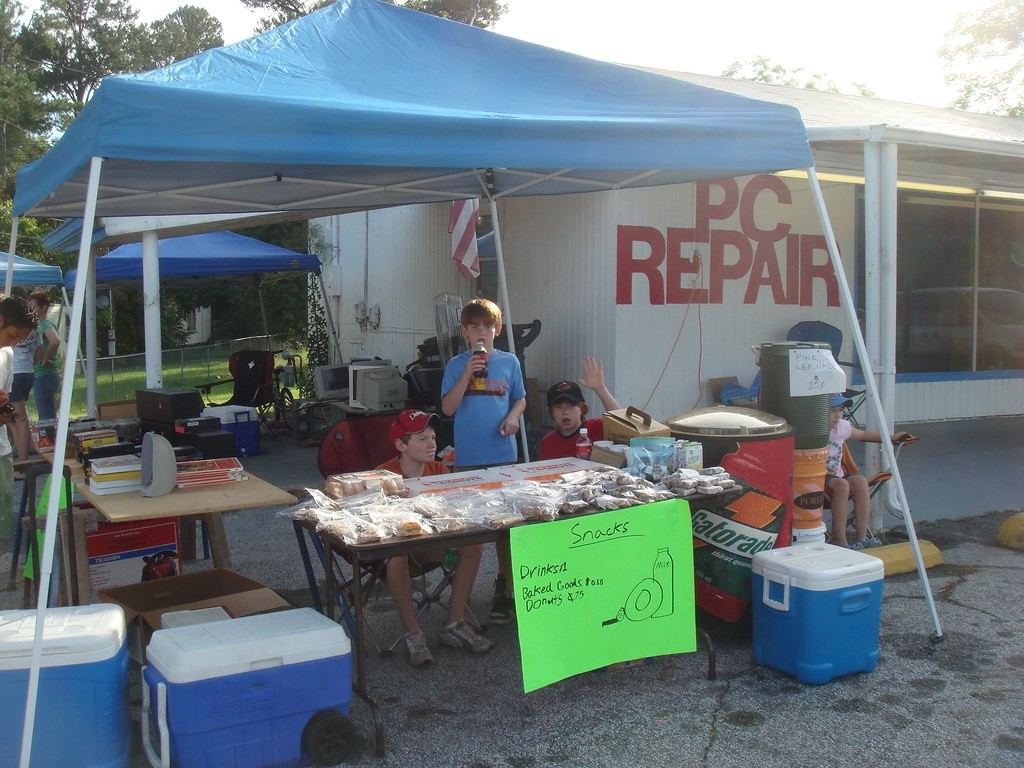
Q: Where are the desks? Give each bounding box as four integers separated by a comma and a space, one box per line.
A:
294, 467, 744, 757
24, 423, 299, 604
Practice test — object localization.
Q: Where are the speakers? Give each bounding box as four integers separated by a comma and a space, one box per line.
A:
140, 432, 178, 497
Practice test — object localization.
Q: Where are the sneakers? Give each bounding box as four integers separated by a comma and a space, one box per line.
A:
440, 619, 495, 653
403, 628, 433, 667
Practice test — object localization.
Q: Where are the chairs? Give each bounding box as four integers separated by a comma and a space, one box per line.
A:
788, 320, 868, 429
409, 364, 455, 435
818, 436, 919, 546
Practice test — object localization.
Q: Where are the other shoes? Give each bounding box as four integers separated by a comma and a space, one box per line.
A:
845, 545, 858, 551
490, 597, 515, 624
854, 538, 870, 549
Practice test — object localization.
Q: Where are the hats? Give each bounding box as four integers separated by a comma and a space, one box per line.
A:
830, 394, 853, 408
547, 381, 581, 406
391, 409, 439, 451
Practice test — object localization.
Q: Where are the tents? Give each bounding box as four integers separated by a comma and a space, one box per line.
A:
0, 0, 943, 768
57, 230, 344, 363
0, 252, 87, 376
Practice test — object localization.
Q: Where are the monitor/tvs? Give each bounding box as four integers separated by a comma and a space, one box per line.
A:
313, 355, 407, 411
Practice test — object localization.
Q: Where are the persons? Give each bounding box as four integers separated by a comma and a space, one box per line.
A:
539, 356, 621, 460
825, 396, 913, 550
0, 297, 39, 556
32, 292, 61, 440
373, 409, 493, 664
441, 299, 527, 624
7, 285, 35, 462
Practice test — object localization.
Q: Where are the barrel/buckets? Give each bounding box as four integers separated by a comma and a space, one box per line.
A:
664, 406, 794, 623
791, 448, 830, 545
627, 436, 676, 485
751, 341, 833, 449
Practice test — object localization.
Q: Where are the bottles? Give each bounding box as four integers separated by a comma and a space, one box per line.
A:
576, 428, 592, 460
283, 359, 294, 387
472, 340, 489, 378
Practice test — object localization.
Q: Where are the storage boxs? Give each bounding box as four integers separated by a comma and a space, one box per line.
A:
750, 542, 885, 684
73, 501, 181, 594
0, 604, 134, 767
98, 567, 290, 662
204, 403, 265, 454
138, 606, 352, 768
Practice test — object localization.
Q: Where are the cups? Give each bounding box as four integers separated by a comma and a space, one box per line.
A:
593, 440, 628, 454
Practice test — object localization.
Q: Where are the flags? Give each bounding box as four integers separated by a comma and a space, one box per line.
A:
449, 198, 480, 280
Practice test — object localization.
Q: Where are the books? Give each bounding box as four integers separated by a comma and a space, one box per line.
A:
74, 429, 141, 495
38, 424, 55, 453
176, 457, 249, 487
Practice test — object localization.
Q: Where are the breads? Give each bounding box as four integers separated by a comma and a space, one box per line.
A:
326, 474, 399, 500
